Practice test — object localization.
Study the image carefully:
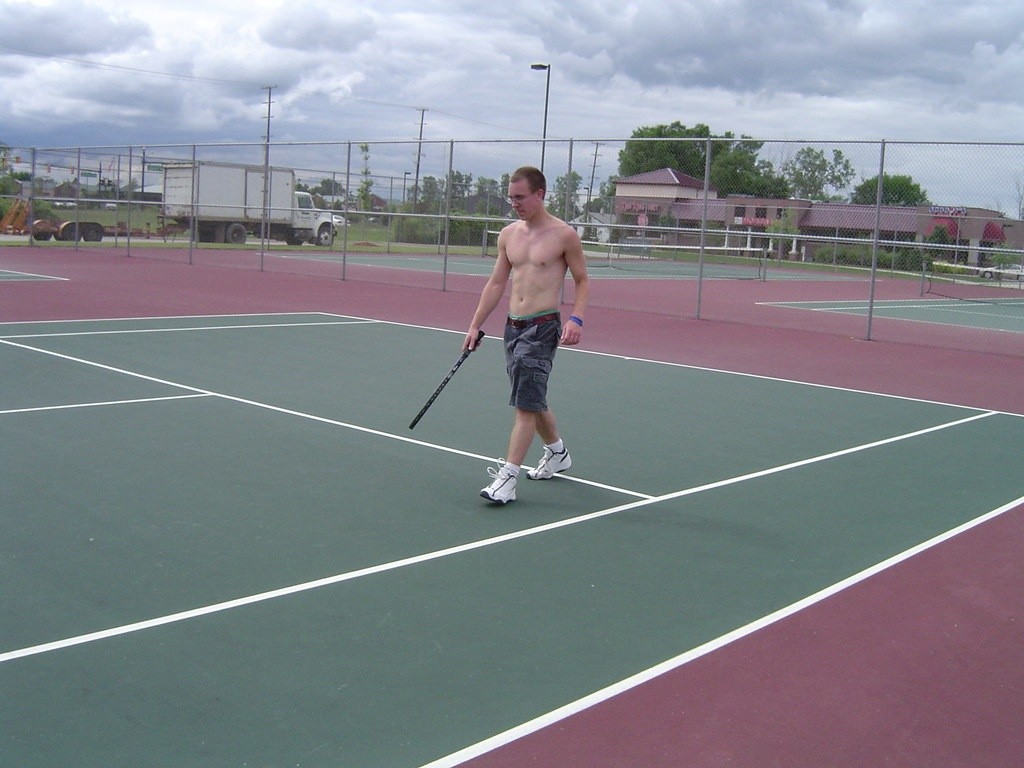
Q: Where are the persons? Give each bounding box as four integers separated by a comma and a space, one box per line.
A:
462, 166, 590, 504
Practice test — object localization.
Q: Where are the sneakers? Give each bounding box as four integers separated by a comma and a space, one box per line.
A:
480, 457, 517, 503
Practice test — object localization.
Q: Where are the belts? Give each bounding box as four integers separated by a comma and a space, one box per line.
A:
506, 312, 560, 328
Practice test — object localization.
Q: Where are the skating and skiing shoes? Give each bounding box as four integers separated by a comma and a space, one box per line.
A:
526, 445, 572, 481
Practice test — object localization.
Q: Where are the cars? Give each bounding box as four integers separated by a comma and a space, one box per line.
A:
332, 215, 351, 228
979, 263, 1024, 283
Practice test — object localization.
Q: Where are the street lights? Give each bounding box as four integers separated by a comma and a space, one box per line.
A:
530, 63, 551, 173
584, 186, 589, 223
403, 172, 412, 202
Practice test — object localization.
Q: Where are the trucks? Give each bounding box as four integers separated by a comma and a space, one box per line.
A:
161, 160, 338, 246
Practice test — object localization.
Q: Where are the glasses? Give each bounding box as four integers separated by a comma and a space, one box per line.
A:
503, 191, 537, 204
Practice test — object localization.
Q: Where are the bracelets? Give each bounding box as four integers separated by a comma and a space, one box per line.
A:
568, 316, 583, 326
